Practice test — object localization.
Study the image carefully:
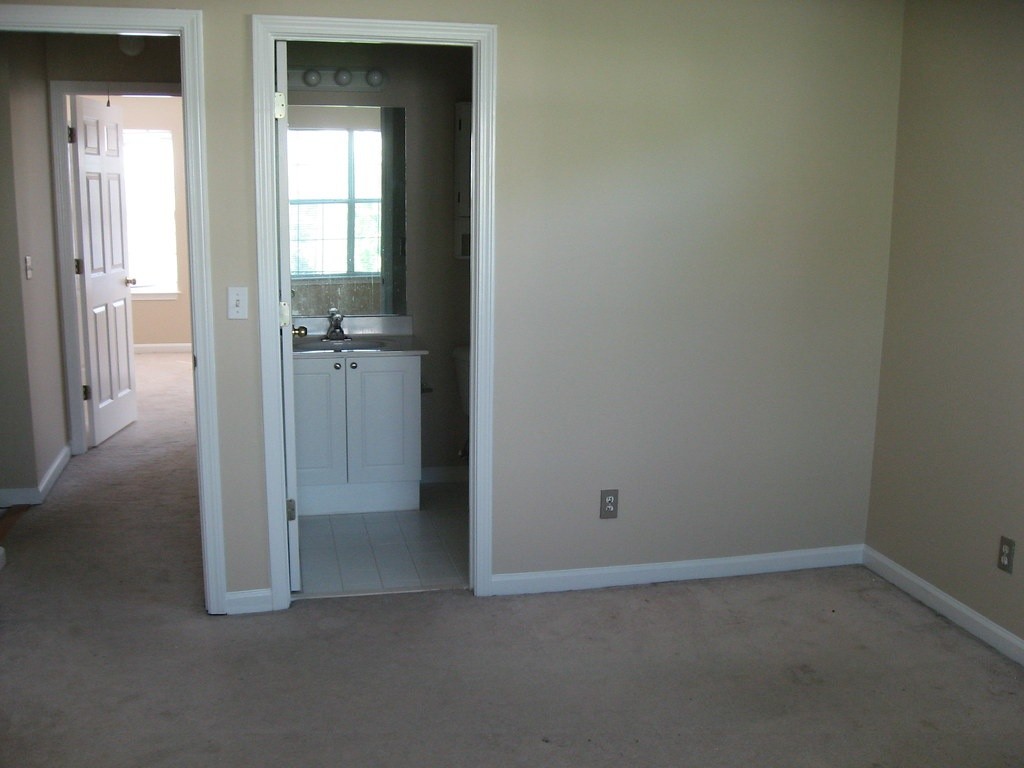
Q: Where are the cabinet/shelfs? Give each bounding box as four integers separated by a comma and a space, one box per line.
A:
294, 353, 421, 517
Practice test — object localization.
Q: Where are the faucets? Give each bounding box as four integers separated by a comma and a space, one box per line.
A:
421, 383, 433, 393
321, 313, 352, 342
328, 307, 338, 315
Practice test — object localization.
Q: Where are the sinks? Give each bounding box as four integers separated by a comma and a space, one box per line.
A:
296, 340, 393, 349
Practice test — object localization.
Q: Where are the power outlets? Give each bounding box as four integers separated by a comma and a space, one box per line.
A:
996, 536, 1016, 573
600, 489, 618, 520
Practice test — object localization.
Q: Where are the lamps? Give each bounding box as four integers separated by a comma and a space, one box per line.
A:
289, 66, 391, 94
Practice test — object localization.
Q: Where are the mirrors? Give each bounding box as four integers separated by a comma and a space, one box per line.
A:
290, 105, 406, 316
